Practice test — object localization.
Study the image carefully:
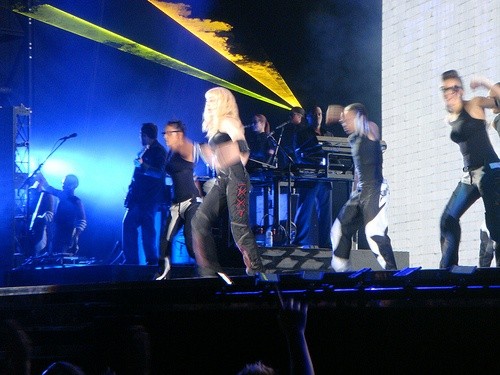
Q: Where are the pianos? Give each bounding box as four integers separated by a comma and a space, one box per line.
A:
301, 135, 387, 157
281, 167, 355, 184
192, 175, 272, 188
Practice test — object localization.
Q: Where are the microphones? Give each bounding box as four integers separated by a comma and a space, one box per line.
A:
267, 131, 275, 139
276, 121, 290, 129
59, 133, 77, 140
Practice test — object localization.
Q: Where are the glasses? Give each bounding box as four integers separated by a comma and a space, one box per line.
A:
439, 86, 462, 93
162, 131, 180, 136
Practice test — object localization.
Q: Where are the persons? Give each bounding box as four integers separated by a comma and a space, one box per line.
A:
292, 106, 334, 249
280, 107, 309, 159
440, 69, 500, 269
190, 88, 273, 294
489, 82, 500, 138
21, 183, 54, 256
122, 123, 167, 266
35, 172, 87, 254
327, 104, 398, 273
248, 114, 275, 167
151, 121, 202, 281
0, 287, 500, 375
